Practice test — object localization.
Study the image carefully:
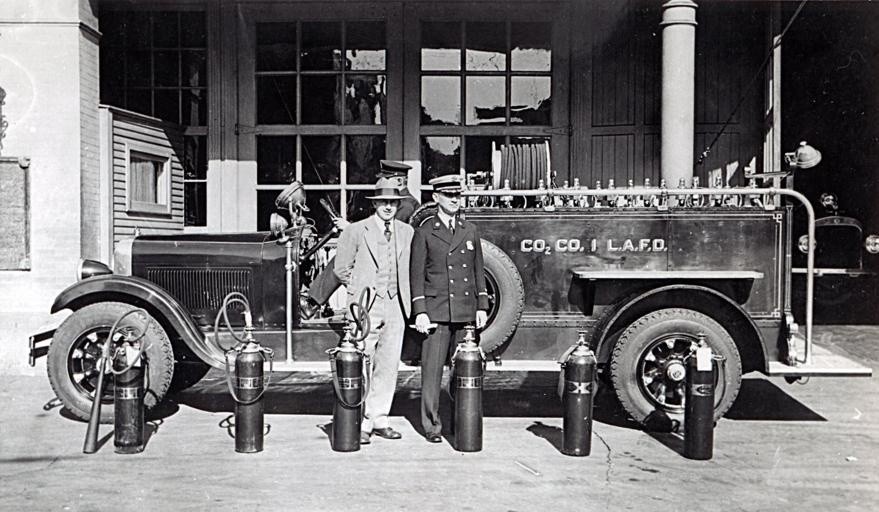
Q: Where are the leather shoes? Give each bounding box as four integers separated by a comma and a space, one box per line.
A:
360, 427, 401, 444
426, 432, 442, 443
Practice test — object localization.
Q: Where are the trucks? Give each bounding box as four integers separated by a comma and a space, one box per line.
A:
28, 175, 821, 427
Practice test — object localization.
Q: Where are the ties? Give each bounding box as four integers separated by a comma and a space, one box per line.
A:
384, 222, 392, 241
449, 220, 455, 235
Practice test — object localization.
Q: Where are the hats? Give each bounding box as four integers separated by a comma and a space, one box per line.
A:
374, 160, 412, 178
429, 174, 465, 194
364, 176, 411, 200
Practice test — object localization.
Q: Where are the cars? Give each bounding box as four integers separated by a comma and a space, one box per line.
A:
785, 169, 879, 322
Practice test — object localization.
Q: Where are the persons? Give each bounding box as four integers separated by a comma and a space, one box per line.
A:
298, 159, 423, 318
330, 176, 417, 444
406, 174, 492, 444
